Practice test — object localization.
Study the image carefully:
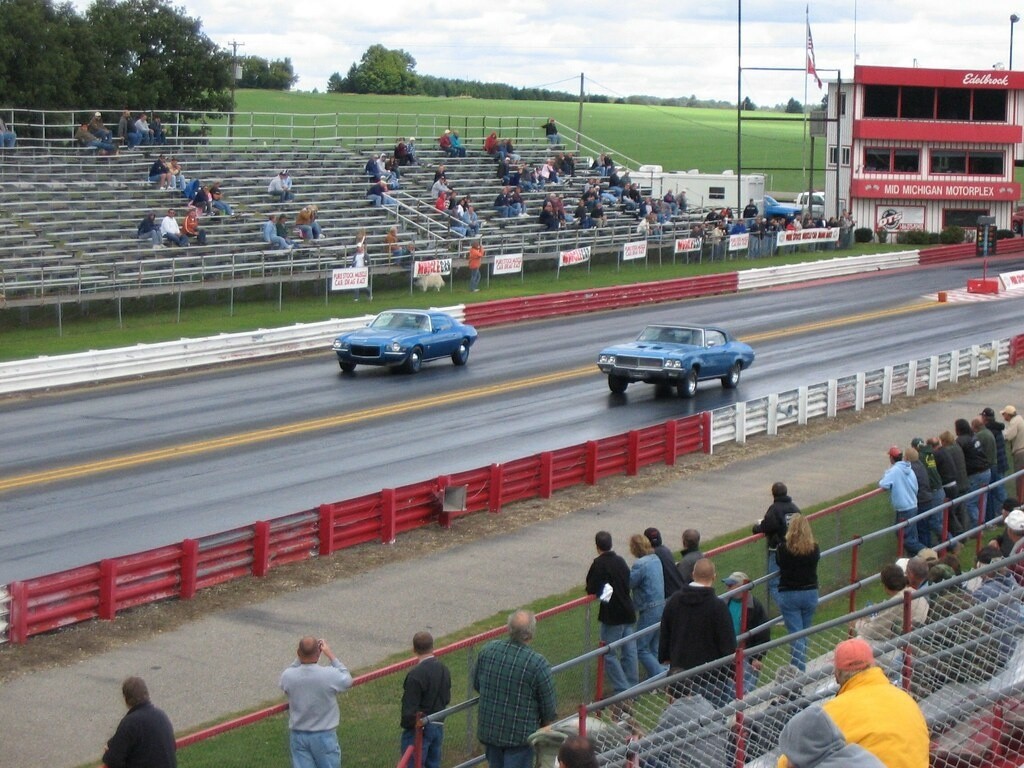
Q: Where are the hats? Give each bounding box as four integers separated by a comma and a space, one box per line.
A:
1000, 405, 1016, 415
1004, 510, 1024, 531
721, 572, 749, 587
834, 639, 872, 670
887, 447, 902, 458
1004, 498, 1021, 512
977, 546, 1002, 564
918, 549, 939, 565
979, 408, 994, 417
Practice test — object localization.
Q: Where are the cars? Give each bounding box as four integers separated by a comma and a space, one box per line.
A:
1012, 206, 1024, 234
763, 194, 802, 222
331, 308, 478, 374
794, 191, 825, 220
596, 320, 757, 399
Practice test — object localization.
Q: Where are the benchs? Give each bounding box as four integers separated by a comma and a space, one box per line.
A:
930, 691, 1024, 768
0, 137, 751, 291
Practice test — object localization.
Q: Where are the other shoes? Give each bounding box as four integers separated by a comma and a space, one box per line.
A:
953, 542, 964, 554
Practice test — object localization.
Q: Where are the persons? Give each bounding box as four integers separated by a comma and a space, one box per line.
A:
413, 316, 426, 329
365, 181, 388, 206
0, 118, 17, 157
471, 608, 557, 768
278, 636, 352, 768
394, 137, 424, 166
484, 118, 687, 240
149, 154, 186, 190
690, 198, 858, 264
688, 331, 702, 345
468, 240, 484, 294
296, 204, 325, 244
268, 169, 293, 208
181, 208, 208, 245
160, 209, 188, 247
348, 244, 373, 302
100, 677, 179, 768
118, 110, 167, 152
187, 178, 235, 216
400, 631, 452, 768
383, 226, 402, 265
137, 212, 168, 249
366, 152, 401, 182
558, 482, 930, 768
879, 405, 1024, 701
263, 213, 300, 250
431, 163, 483, 237
75, 112, 118, 156
401, 243, 415, 268
439, 129, 467, 158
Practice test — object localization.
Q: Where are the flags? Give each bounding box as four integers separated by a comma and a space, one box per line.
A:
808, 26, 822, 90
